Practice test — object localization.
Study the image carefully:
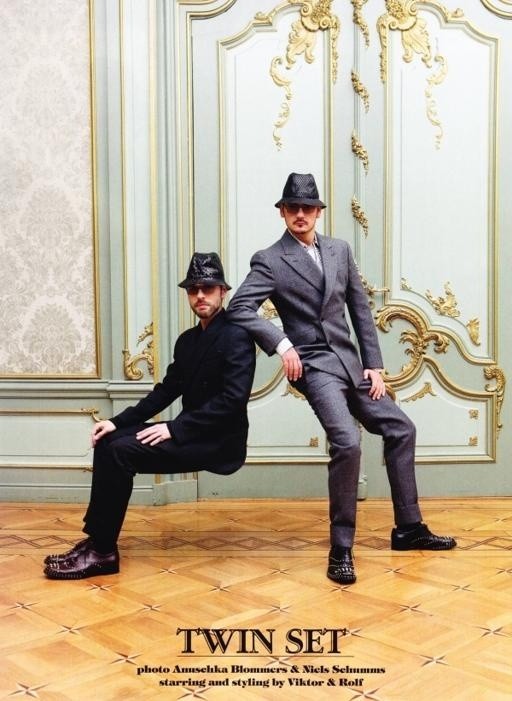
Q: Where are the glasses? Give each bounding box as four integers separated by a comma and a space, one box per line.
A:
185, 286, 214, 295
286, 203, 315, 215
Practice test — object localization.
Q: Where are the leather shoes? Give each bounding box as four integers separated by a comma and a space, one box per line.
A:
44, 538, 120, 580
327, 547, 357, 584
390, 523, 457, 551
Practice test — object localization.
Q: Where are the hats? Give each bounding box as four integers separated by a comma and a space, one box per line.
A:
274, 173, 327, 208
178, 251, 234, 291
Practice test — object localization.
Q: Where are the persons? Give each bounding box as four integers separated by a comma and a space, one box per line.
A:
227, 173, 457, 584
42, 253, 256, 579
